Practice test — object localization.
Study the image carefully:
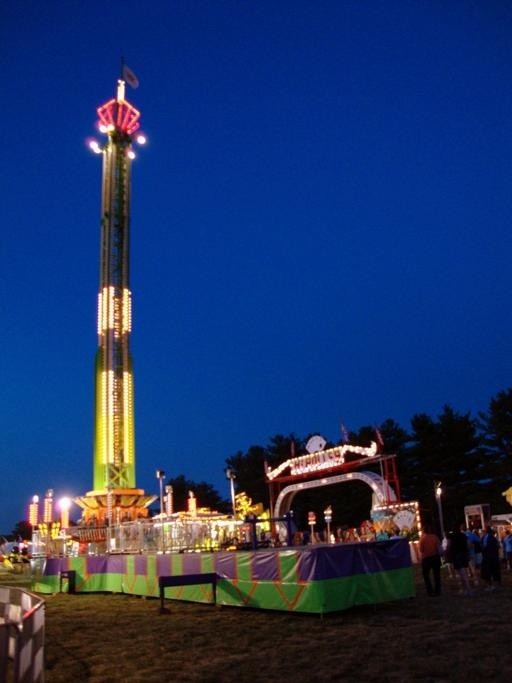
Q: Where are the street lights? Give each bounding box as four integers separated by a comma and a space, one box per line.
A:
163, 484, 174, 515
44, 487, 55, 528
156, 469, 167, 523
30, 492, 39, 530
58, 496, 72, 528
186, 488, 198, 514
432, 480, 448, 538
225, 468, 238, 517
267, 465, 273, 519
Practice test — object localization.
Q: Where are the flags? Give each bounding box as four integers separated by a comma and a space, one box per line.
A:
122, 65, 140, 90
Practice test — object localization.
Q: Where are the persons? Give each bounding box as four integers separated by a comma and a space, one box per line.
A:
417, 520, 512, 598
